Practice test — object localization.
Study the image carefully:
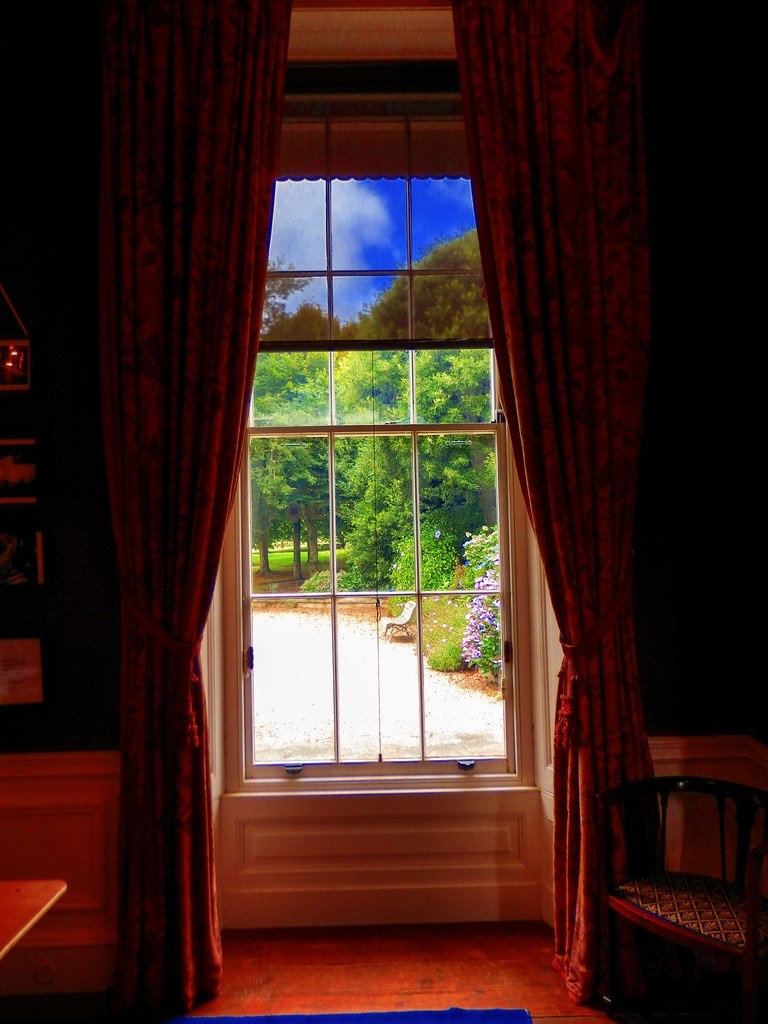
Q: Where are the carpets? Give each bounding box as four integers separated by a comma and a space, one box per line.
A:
165, 1008, 534, 1024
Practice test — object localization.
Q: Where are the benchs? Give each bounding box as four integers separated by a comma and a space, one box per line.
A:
379, 600, 417, 639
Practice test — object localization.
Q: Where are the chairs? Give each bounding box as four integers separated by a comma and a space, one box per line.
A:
595, 776, 768, 1024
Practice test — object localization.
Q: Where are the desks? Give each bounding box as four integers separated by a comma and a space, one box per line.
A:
0, 878, 69, 961
